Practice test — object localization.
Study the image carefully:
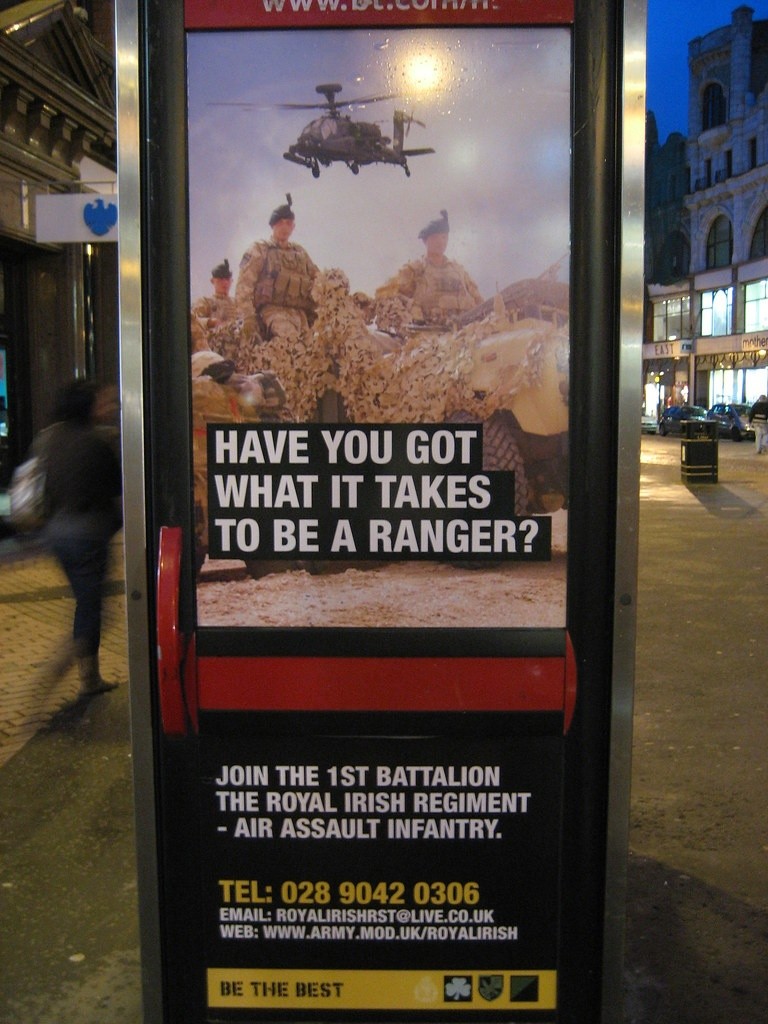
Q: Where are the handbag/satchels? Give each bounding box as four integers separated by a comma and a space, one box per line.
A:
5, 422, 65, 527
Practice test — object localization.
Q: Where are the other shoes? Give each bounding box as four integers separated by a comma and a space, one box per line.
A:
79, 680, 118, 695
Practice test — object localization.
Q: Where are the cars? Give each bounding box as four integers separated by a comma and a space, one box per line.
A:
659, 406, 709, 440
706, 403, 756, 443
641, 416, 658, 435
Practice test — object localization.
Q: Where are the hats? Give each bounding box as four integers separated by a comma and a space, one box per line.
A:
418, 209, 450, 239
269, 192, 295, 228
212, 258, 232, 278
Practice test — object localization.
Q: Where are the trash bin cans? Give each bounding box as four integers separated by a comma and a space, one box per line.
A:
679, 419, 719, 482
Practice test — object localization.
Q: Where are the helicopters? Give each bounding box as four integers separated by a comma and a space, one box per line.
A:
205, 84, 436, 179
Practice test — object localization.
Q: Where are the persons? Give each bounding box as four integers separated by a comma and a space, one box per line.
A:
33, 376, 120, 701
193, 265, 235, 339
236, 209, 318, 346
388, 213, 472, 302
